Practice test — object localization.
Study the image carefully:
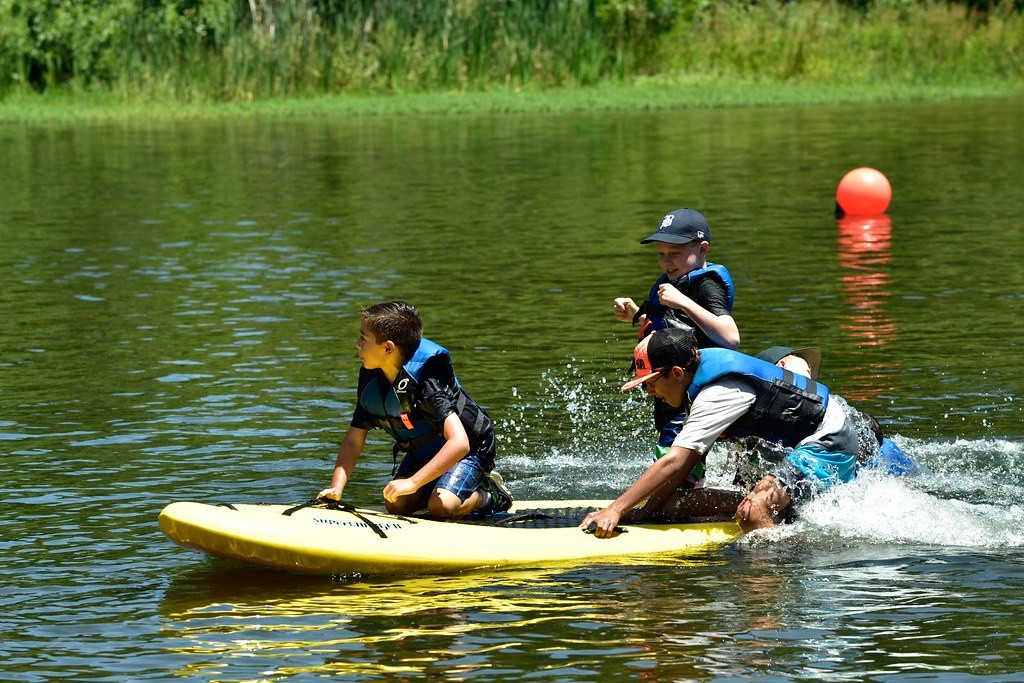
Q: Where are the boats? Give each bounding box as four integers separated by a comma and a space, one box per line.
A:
157, 496, 802, 575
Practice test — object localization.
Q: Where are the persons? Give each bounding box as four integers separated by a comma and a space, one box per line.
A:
317, 301, 514, 519
579, 208, 935, 539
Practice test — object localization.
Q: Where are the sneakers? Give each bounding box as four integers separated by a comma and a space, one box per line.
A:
479, 471, 514, 512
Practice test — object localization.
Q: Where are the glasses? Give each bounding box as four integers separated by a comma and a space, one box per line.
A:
643, 366, 685, 391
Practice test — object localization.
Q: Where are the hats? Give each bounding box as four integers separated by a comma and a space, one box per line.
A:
640, 208, 710, 245
755, 345, 822, 381
621, 328, 696, 391
